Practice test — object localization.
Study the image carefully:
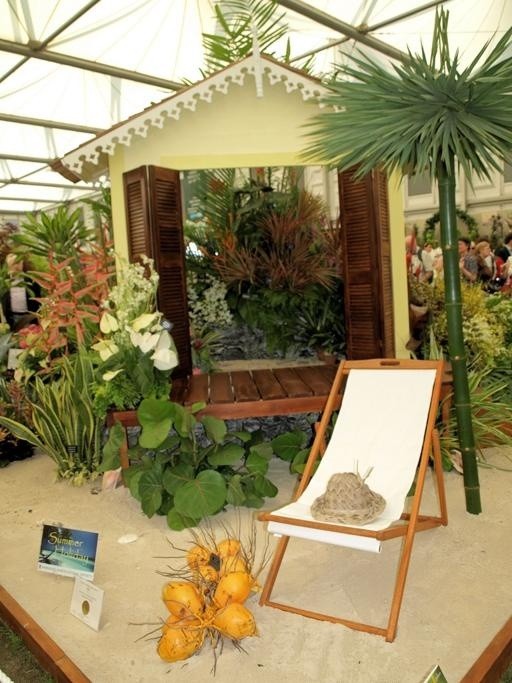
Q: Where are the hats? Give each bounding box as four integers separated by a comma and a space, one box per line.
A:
310, 459, 387, 525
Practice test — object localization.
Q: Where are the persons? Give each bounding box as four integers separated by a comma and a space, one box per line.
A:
406, 232, 512, 301
1, 253, 42, 335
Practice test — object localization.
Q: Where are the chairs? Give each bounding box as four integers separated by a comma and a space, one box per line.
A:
259, 358, 447, 642
